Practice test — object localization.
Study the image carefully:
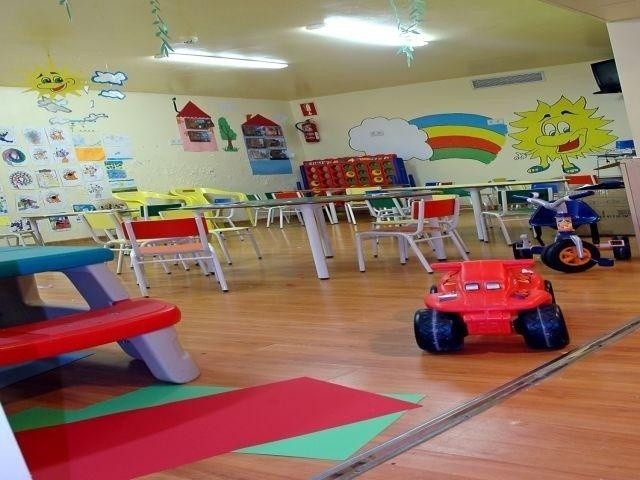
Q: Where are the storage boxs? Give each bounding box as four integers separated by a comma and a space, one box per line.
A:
568, 189, 636, 236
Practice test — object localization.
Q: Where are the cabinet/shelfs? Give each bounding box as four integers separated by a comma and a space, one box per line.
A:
300, 153, 407, 189
